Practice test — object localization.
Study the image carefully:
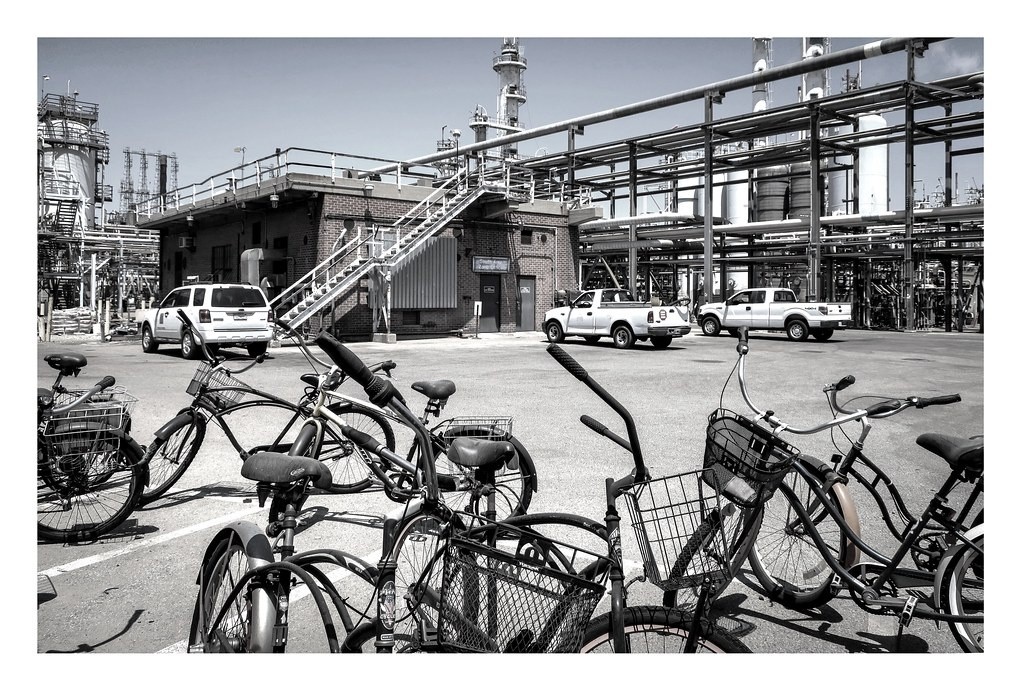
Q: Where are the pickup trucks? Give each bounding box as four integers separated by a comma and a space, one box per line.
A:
538, 286, 692, 348
698, 286, 853, 343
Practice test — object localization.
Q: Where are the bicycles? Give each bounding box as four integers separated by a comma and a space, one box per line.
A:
39, 349, 980, 654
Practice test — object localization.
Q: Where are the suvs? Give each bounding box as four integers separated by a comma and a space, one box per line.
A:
139, 280, 276, 361
